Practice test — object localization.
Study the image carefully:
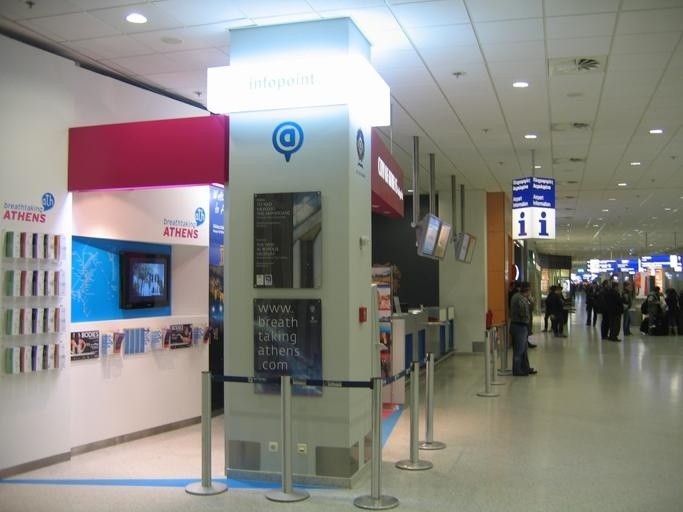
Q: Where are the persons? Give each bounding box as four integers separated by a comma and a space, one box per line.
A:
506, 278, 682, 349
379, 332, 391, 379
176, 327, 191, 344
134, 272, 164, 296
377, 293, 390, 309
71, 338, 87, 354
509, 281, 538, 377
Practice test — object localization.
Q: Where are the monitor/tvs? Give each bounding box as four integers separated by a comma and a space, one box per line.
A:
464, 235, 478, 260
417, 214, 440, 260
457, 232, 469, 263
433, 223, 453, 263
120, 251, 168, 309
